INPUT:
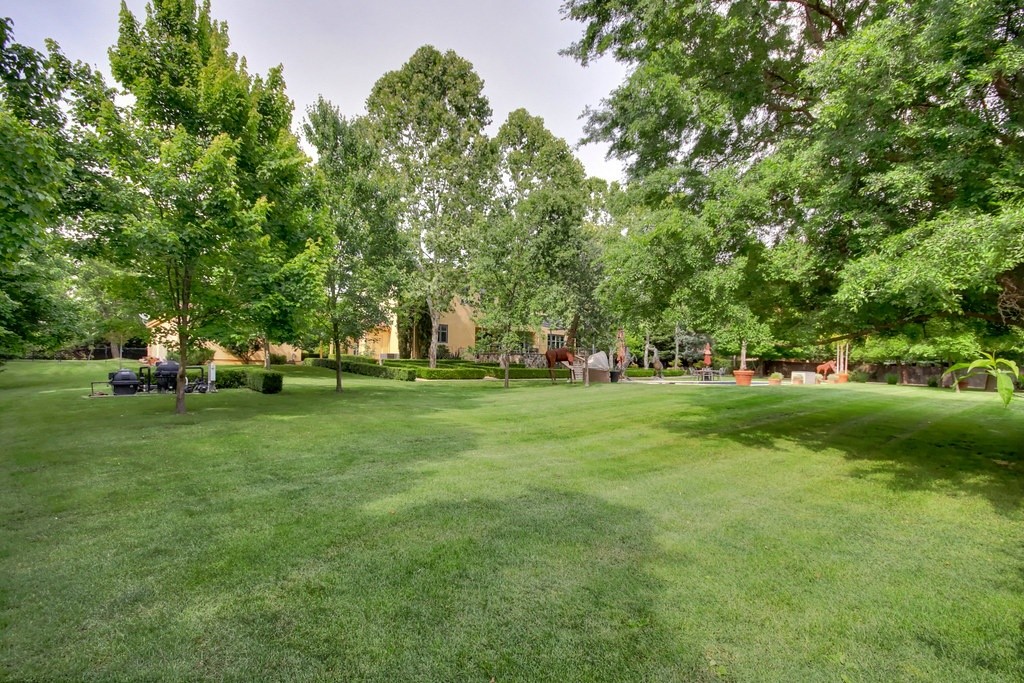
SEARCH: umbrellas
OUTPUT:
[704,343,712,368]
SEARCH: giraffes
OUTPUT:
[546,326,577,382]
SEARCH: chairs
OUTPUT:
[692,367,722,381]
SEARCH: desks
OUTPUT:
[693,370,719,380]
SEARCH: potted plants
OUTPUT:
[793,374,804,385]
[837,369,848,382]
[769,372,784,385]
[815,374,823,384]
[609,364,620,383]
